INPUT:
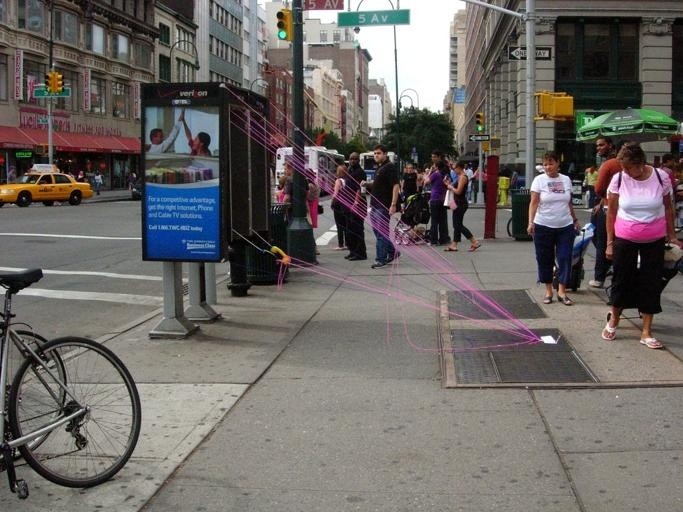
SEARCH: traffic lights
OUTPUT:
[54,72,64,93]
[490,137,501,149]
[45,73,53,94]
[276,8,294,41]
[536,93,552,117]
[475,112,485,133]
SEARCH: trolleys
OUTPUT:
[605,240,682,330]
[553,229,586,294]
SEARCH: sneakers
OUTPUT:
[344,254,366,260]
[333,246,348,249]
[387,250,400,263]
[371,261,384,267]
[589,280,603,287]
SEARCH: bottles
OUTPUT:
[360,183,366,197]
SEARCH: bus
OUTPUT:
[272,145,345,197]
[359,151,398,196]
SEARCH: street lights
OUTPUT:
[249,78,273,98]
[167,40,200,84]
[398,88,420,113]
[353,1,399,123]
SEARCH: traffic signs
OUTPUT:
[467,135,491,142]
[506,44,553,62]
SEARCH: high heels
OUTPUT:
[544,293,553,303]
[557,292,573,305]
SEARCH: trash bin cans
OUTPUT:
[510,189,532,241]
[246,204,289,284]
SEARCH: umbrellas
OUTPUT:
[575,106,682,142]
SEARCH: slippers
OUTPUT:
[467,244,480,251]
[602,311,616,340]
[640,338,663,349]
[444,247,457,251]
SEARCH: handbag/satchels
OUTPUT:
[443,189,454,207]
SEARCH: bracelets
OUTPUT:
[606,241,613,246]
[668,238,678,244]
[573,218,577,223]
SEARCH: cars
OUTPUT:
[130,177,142,201]
[0,163,94,208]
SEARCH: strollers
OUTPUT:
[394,182,434,246]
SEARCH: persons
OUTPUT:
[0,163,139,206]
[527,149,584,307]
[601,141,682,351]
[583,138,683,288]
[179,108,218,158]
[146,108,179,158]
[330,145,485,268]
[500,164,519,189]
[277,160,317,229]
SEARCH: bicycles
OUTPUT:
[1,311,67,464]
[0,268,142,499]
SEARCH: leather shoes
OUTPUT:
[606,287,612,305]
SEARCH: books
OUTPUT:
[146,163,216,185]
